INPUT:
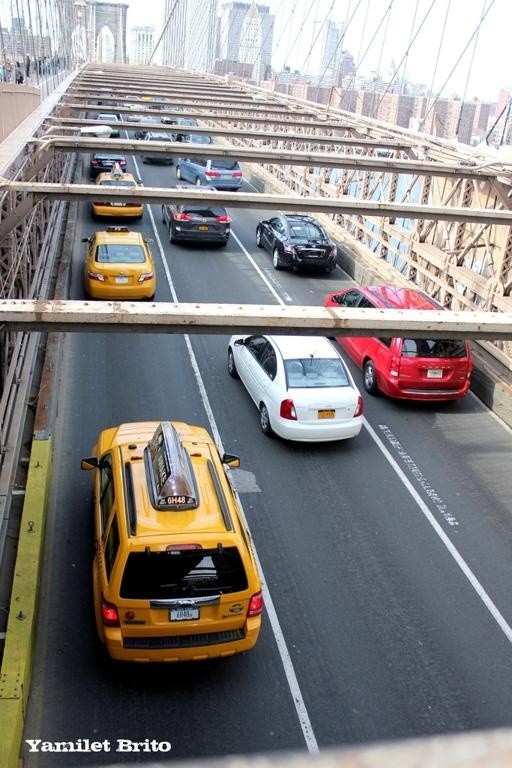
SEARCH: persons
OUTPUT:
[0,52,60,85]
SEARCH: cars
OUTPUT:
[256,215,337,273]
[91,93,243,245]
[81,226,156,301]
[81,421,262,663]
[228,285,474,443]
